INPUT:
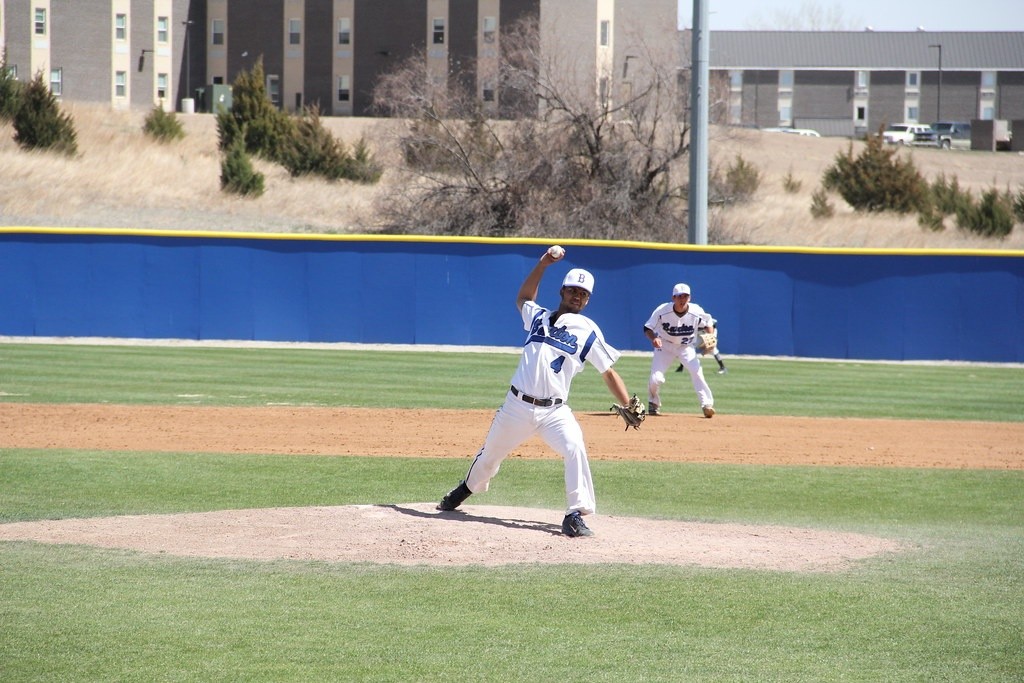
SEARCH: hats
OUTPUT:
[561,269,595,294]
[672,283,690,296]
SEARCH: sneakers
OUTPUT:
[562,511,596,539]
[440,481,471,511]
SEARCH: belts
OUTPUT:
[511,385,563,407]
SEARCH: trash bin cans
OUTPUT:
[205,84,233,114]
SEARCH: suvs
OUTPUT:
[913,121,972,152]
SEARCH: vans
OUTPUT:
[871,122,932,146]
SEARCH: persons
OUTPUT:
[675,317,726,373]
[437,249,645,538]
[644,283,716,418]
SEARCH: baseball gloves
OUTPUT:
[698,333,717,356]
[609,393,646,431]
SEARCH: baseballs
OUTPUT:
[551,245,562,258]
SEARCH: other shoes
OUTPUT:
[702,405,714,418]
[648,401,660,415]
[717,368,727,374]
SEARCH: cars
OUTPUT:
[761,127,820,137]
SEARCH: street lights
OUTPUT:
[182,20,194,98]
[928,43,942,123]
